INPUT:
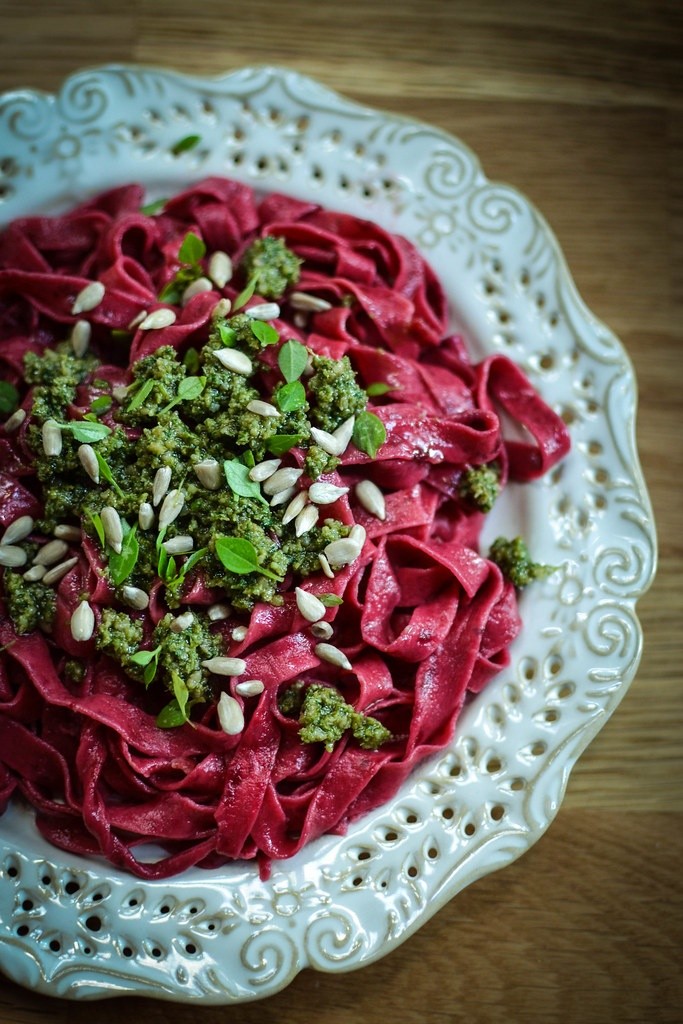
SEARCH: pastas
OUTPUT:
[0,178,572,877]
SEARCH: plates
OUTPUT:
[0,62,658,1000]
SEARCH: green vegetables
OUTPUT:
[54,201,397,728]
